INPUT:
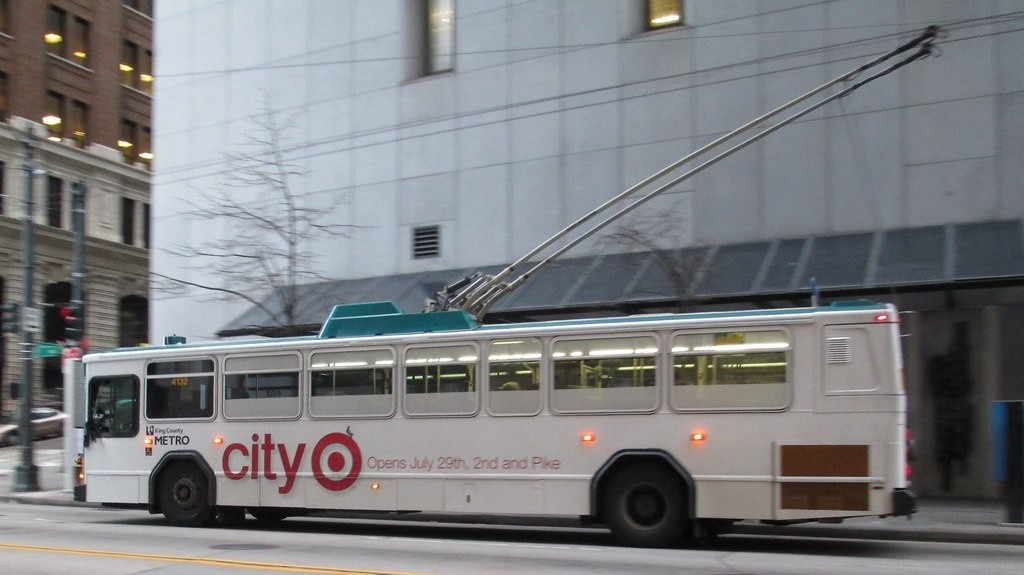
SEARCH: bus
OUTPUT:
[70,24,944,551]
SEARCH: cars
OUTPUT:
[0,407,65,449]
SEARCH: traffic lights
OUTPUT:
[59,304,85,343]
[0,302,18,335]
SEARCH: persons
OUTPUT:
[924,344,974,495]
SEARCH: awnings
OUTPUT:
[213,214,1024,341]
